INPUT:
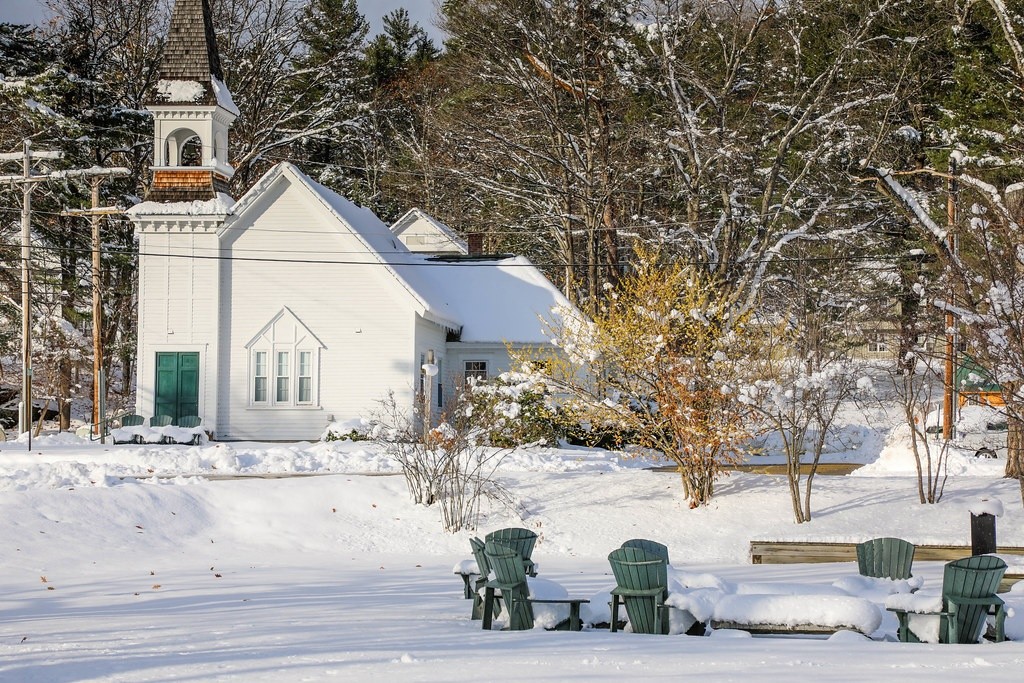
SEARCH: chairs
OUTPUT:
[621,539,669,567]
[483,542,590,631]
[169,416,201,445]
[842,537,921,596]
[610,547,677,635]
[112,416,144,443]
[140,415,172,443]
[888,556,1008,644]
[454,537,501,619]
[484,528,537,577]
